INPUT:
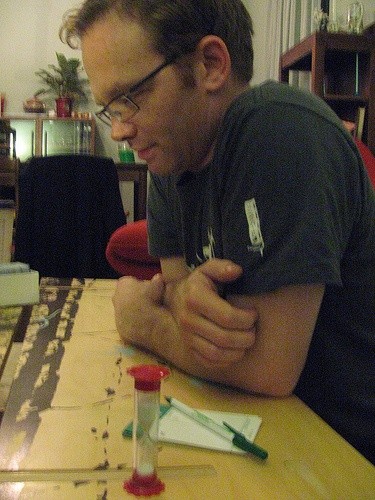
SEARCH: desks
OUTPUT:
[0,277,375,500]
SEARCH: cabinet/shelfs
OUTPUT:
[277,29,368,142]
[0,111,149,263]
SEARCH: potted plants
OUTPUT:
[34,52,89,117]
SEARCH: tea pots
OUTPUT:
[21,96,47,113]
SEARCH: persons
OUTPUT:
[59,0,375,466]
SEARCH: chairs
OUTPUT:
[14,152,126,279]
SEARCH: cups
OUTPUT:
[71,111,89,119]
[118,142,134,164]
[347,1,363,35]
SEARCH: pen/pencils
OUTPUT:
[164,394,269,461]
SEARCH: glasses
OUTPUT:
[94,59,173,127]
